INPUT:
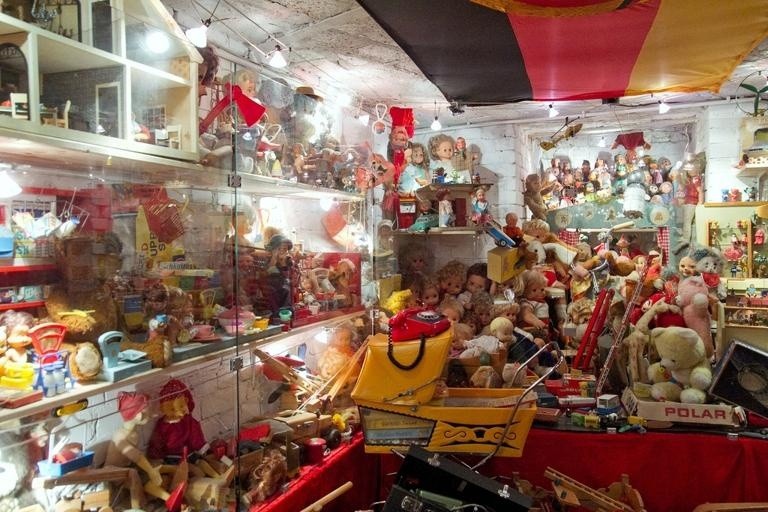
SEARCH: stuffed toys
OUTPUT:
[0,45,767,512]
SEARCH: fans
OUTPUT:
[735,70,768,116]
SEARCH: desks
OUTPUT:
[379,411,768,512]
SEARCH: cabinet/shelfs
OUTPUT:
[704,164,768,365]
[393,182,492,263]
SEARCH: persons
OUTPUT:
[0,45,767,512]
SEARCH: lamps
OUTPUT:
[354,111,371,128]
[656,98,671,115]
[596,136,608,150]
[431,101,442,131]
[184,1,224,49]
[267,43,288,69]
[548,102,560,119]
